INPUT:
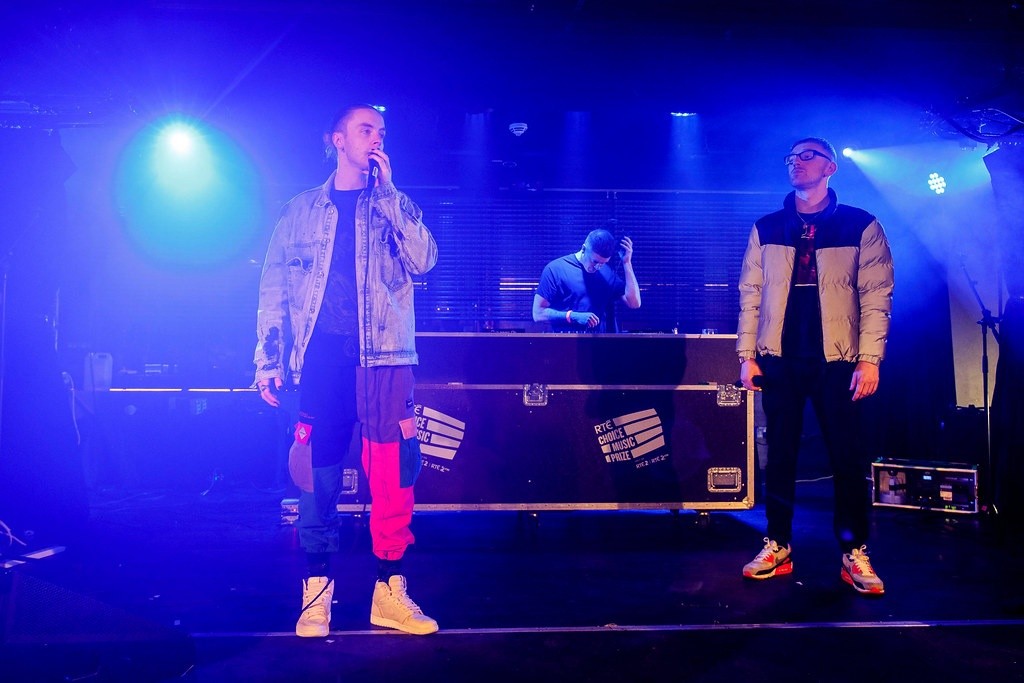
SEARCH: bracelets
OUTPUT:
[566,311,573,323]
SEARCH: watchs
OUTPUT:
[739,356,753,364]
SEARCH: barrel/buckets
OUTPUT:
[84,351,113,391]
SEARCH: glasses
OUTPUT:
[784,150,832,167]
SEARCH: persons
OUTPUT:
[249,104,439,638]
[532,229,641,333]
[735,136,895,594]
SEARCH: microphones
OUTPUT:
[735,376,763,387]
[366,153,380,200]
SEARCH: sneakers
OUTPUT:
[743,537,794,580]
[369,574,439,636]
[840,545,885,595]
[294,575,333,637]
